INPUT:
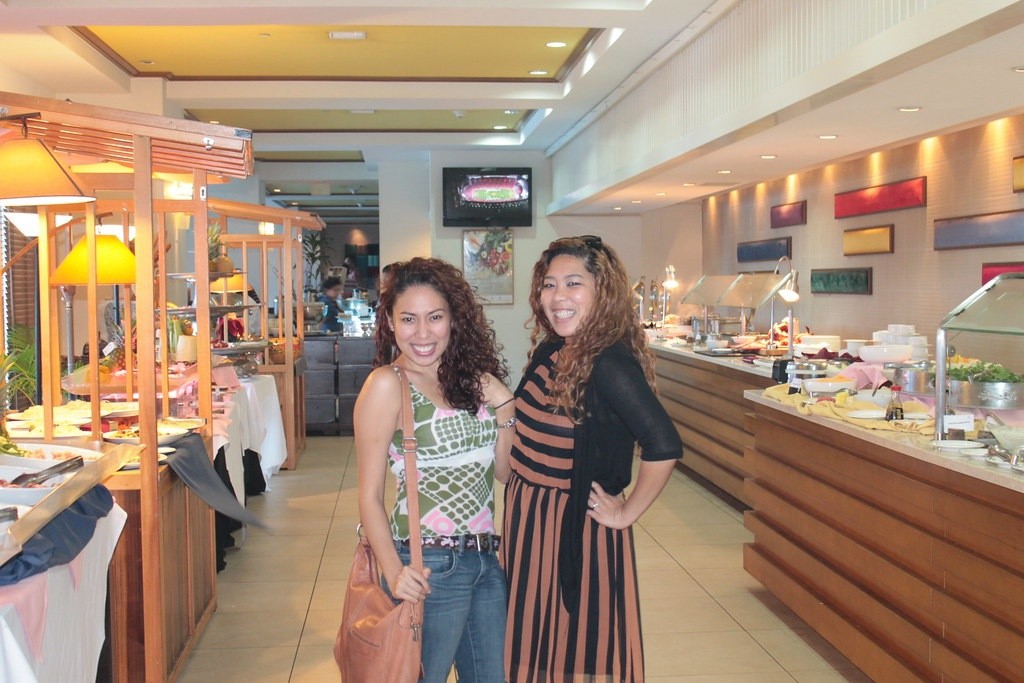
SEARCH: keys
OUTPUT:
[410,621,420,642]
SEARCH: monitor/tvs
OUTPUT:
[442,167,533,227]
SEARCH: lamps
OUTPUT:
[2,210,136,286]
[258,221,275,235]
[209,270,253,294]
[767,255,800,349]
[0,111,97,206]
[662,264,679,332]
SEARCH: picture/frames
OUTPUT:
[462,229,514,305]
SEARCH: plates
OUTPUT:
[157,446,176,453]
[931,439,1024,471]
[802,390,932,420]
[122,453,168,469]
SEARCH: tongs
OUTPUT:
[988,443,1020,464]
[162,417,207,428]
[12,455,85,486]
[0,508,18,524]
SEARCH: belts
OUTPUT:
[400,532,501,552]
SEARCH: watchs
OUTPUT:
[497,417,516,428]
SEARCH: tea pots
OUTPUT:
[355,303,373,317]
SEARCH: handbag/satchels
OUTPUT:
[333,536,425,683]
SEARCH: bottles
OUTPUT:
[885,386,904,420]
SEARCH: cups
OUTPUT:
[269,318,286,337]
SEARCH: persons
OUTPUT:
[352,258,517,683]
[504,235,685,683]
[315,276,345,333]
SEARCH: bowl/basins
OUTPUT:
[641,315,929,392]
[0,400,204,530]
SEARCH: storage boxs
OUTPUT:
[302,336,377,424]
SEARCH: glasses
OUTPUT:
[554,234,605,253]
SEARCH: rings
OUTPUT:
[592,504,600,509]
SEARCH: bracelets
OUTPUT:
[494,398,515,411]
